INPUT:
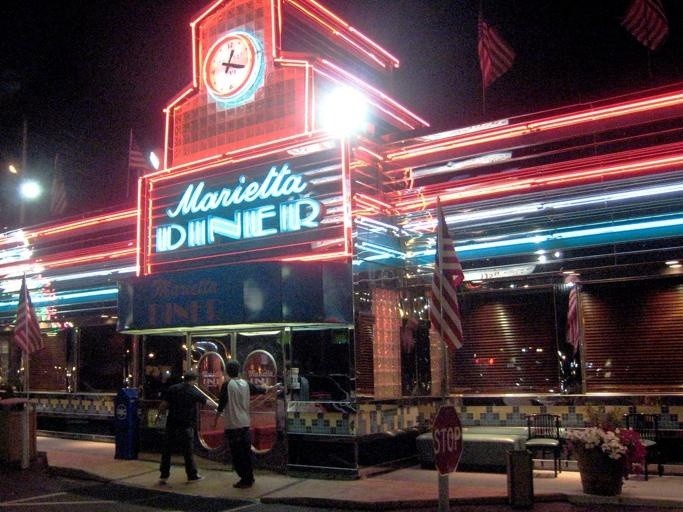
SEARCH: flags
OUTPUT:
[429,203,466,351]
[618,0,670,52]
[476,11,515,89]
[564,282,582,358]
[128,130,153,176]
[12,275,45,357]
[48,159,68,219]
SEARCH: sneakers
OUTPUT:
[232,476,257,489]
[157,472,206,486]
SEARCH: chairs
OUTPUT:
[523,414,563,478]
[623,413,664,480]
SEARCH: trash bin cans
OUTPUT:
[111,387,141,460]
[0,398,38,470]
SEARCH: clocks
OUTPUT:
[200,31,266,108]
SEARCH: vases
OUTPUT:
[575,441,623,497]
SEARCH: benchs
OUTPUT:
[416,434,524,472]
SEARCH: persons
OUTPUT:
[156,370,218,485]
[258,364,285,401]
[279,358,311,402]
[211,360,274,488]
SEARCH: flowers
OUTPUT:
[562,414,648,480]
